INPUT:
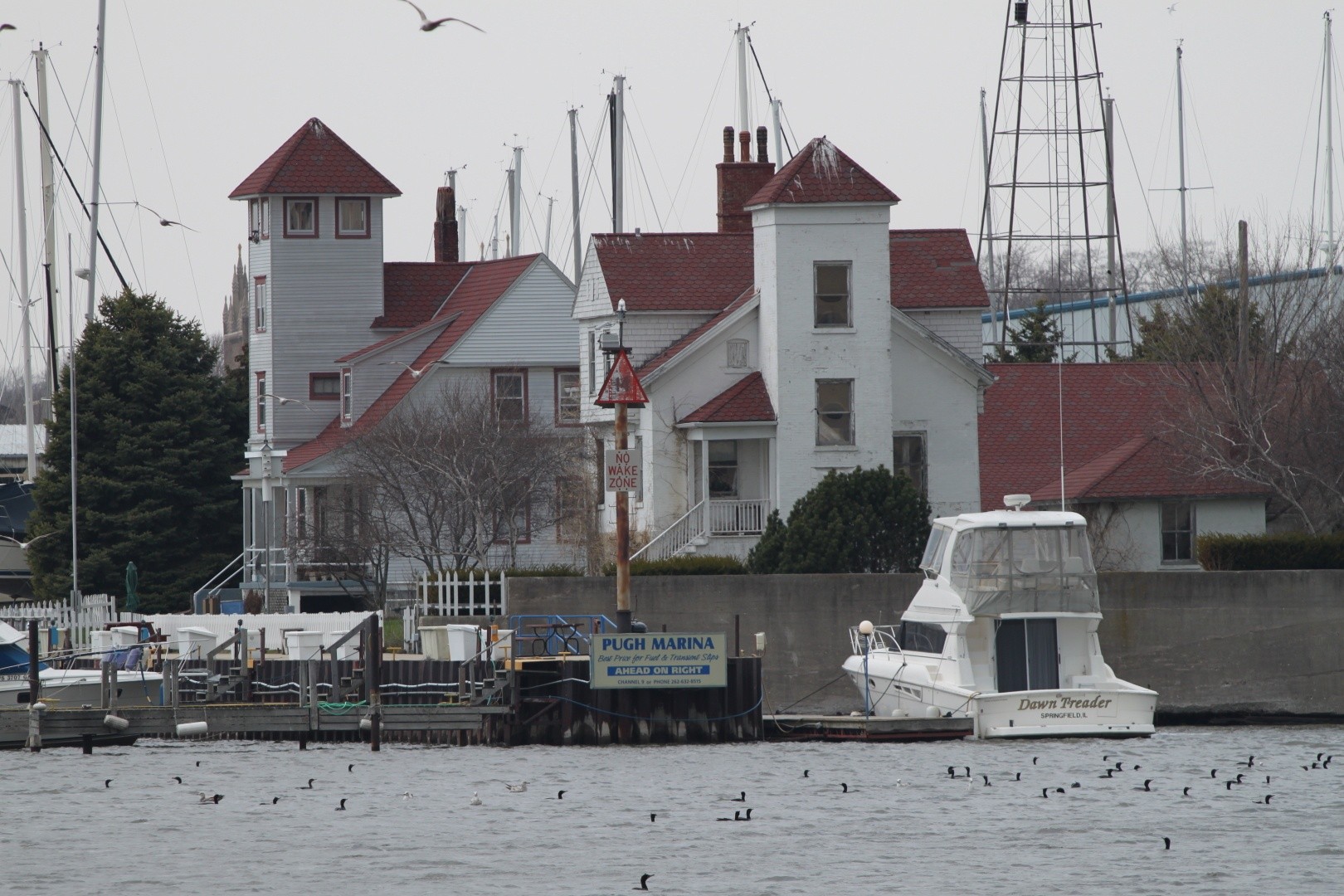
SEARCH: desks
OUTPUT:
[526,624,585,657]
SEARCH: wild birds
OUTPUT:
[1,731,1343,895]
[245,392,311,412]
[401,0,484,34]
[375,360,450,380]
[0,526,66,551]
[137,204,197,233]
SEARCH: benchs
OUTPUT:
[516,637,548,657]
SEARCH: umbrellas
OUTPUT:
[125,562,140,612]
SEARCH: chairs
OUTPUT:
[1058,556,1087,588]
[1013,556,1041,588]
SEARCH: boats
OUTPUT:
[0,617,211,748]
[838,492,1162,736]
